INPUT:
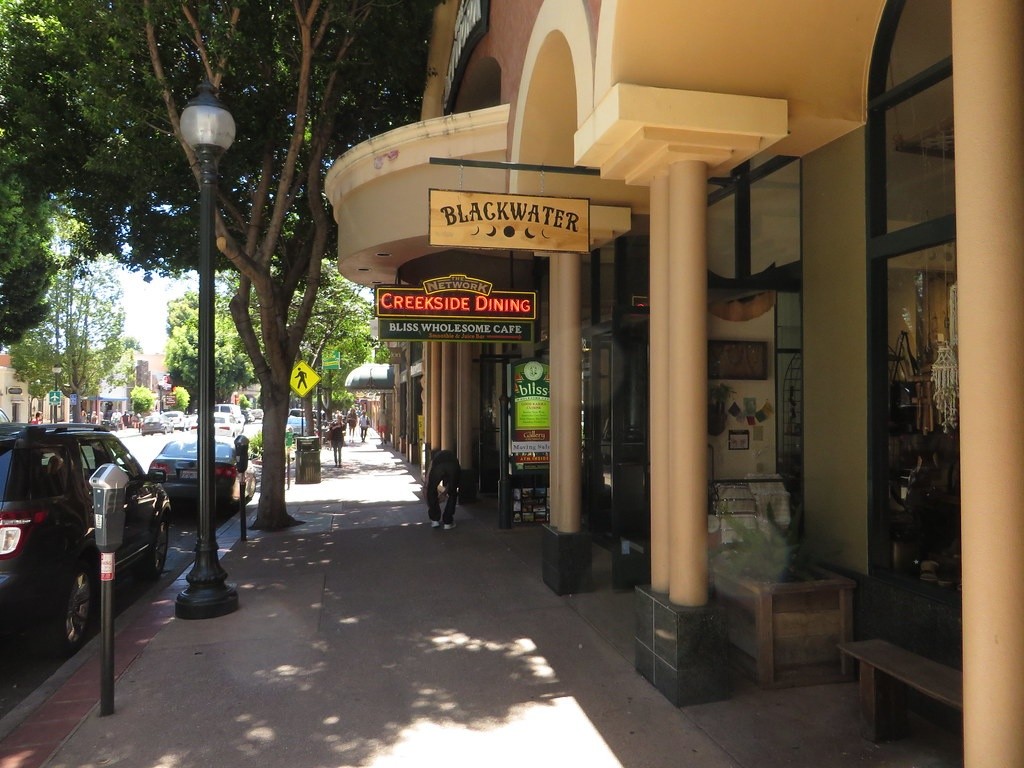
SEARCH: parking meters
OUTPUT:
[286,425,293,490]
[234,435,250,542]
[89,462,131,717]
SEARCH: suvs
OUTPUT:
[0,424,172,653]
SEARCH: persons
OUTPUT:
[82,410,98,424]
[347,408,371,442]
[378,412,388,444]
[100,408,130,430]
[330,415,346,468]
[425,450,461,529]
[32,412,42,424]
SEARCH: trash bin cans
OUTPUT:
[295,437,321,484]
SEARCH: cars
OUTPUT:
[215,403,264,437]
[142,411,198,436]
[287,409,317,439]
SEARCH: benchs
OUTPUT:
[834,639,963,743]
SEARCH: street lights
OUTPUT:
[158,378,165,414]
[52,364,63,423]
[174,79,240,619]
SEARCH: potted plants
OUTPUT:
[709,383,737,416]
[708,495,857,691]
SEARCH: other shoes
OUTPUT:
[444,523,456,530]
[431,521,440,527]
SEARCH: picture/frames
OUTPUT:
[707,339,768,380]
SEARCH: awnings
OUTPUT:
[345,367,395,390]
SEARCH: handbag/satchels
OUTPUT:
[366,417,370,425]
[329,427,333,440]
